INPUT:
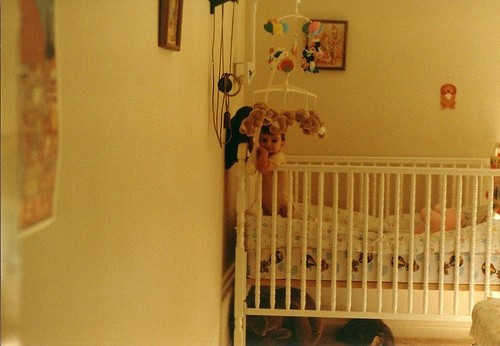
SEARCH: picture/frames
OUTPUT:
[159,0,182,51]
[308,19,348,70]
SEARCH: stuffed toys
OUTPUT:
[248,287,323,345]
[239,103,326,139]
[419,205,464,234]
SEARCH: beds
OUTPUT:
[232,143,500,346]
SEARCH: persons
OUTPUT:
[259,126,294,217]
